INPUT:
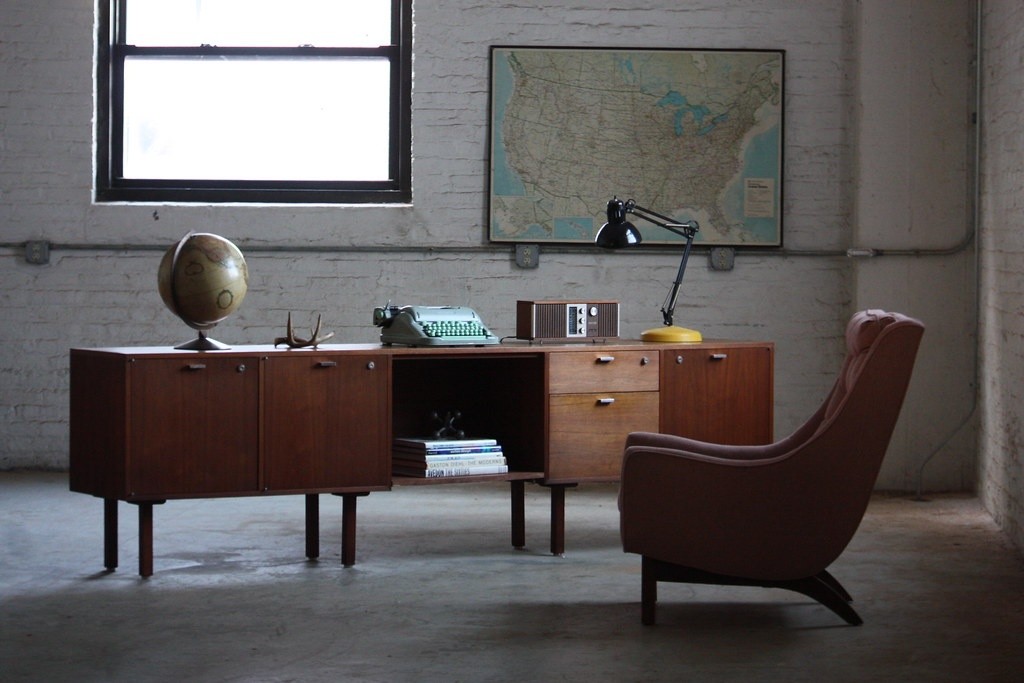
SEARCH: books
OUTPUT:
[392,438,508,478]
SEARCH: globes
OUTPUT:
[156,228,249,351]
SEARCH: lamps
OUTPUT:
[596,196,702,342]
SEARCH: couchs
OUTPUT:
[617,308,926,627]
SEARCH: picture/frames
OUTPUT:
[488,45,785,247]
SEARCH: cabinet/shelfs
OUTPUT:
[69,346,259,501]
[545,339,660,482]
[660,339,775,446]
[261,345,391,494]
